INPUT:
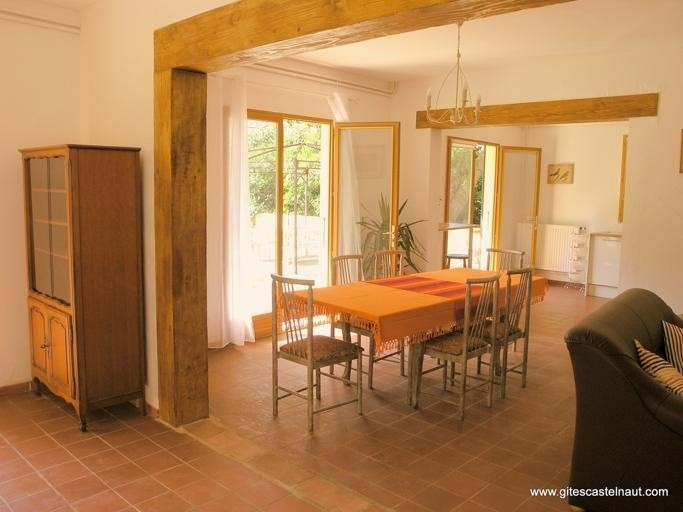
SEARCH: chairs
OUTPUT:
[405,273,503,421]
[486,246,522,272]
[565,287,682,510]
[268,272,366,431]
[374,251,406,280]
[331,254,406,388]
[449,267,532,399]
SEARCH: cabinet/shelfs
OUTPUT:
[17,144,148,432]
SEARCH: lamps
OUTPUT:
[423,13,485,130]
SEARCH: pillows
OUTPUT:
[657,318,683,372]
[631,333,682,396]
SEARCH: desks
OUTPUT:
[443,254,468,266]
[277,265,548,416]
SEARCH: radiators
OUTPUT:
[517,223,581,271]
